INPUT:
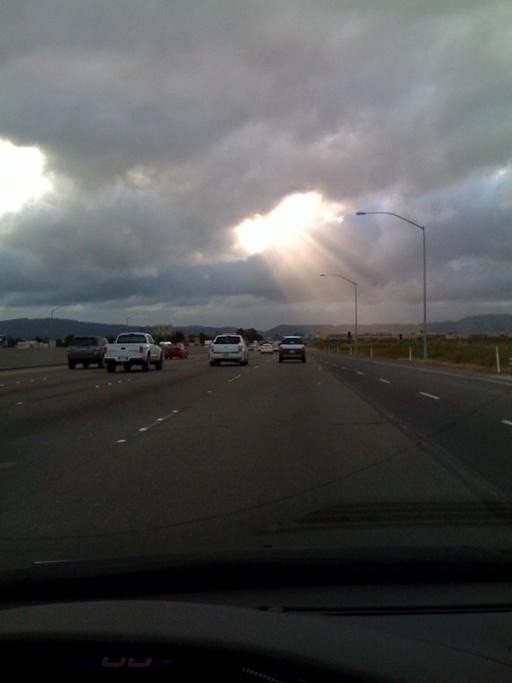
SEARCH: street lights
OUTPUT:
[49,302,78,338]
[318,272,359,342]
[354,210,429,361]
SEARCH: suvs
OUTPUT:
[279,335,307,363]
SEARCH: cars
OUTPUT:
[162,341,190,359]
[158,340,172,347]
[248,337,282,354]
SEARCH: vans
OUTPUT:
[66,334,111,370]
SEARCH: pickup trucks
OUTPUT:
[103,331,163,372]
[207,333,250,366]
